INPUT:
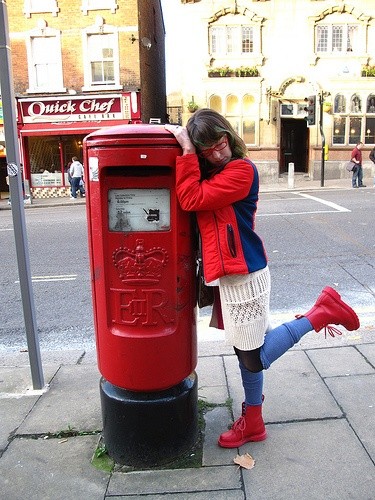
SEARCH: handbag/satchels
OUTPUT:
[346,162,355,172]
[197,261,215,308]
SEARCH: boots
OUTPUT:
[295,286,361,335]
[217,394,267,448]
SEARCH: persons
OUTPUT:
[164,108,360,448]
[369,147,375,163]
[350,142,366,188]
[67,156,86,200]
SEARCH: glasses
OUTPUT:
[198,136,228,158]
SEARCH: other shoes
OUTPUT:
[81,192,86,197]
[352,184,358,188]
[358,184,366,187]
[70,194,78,200]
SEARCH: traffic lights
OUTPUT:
[303,95,317,126]
